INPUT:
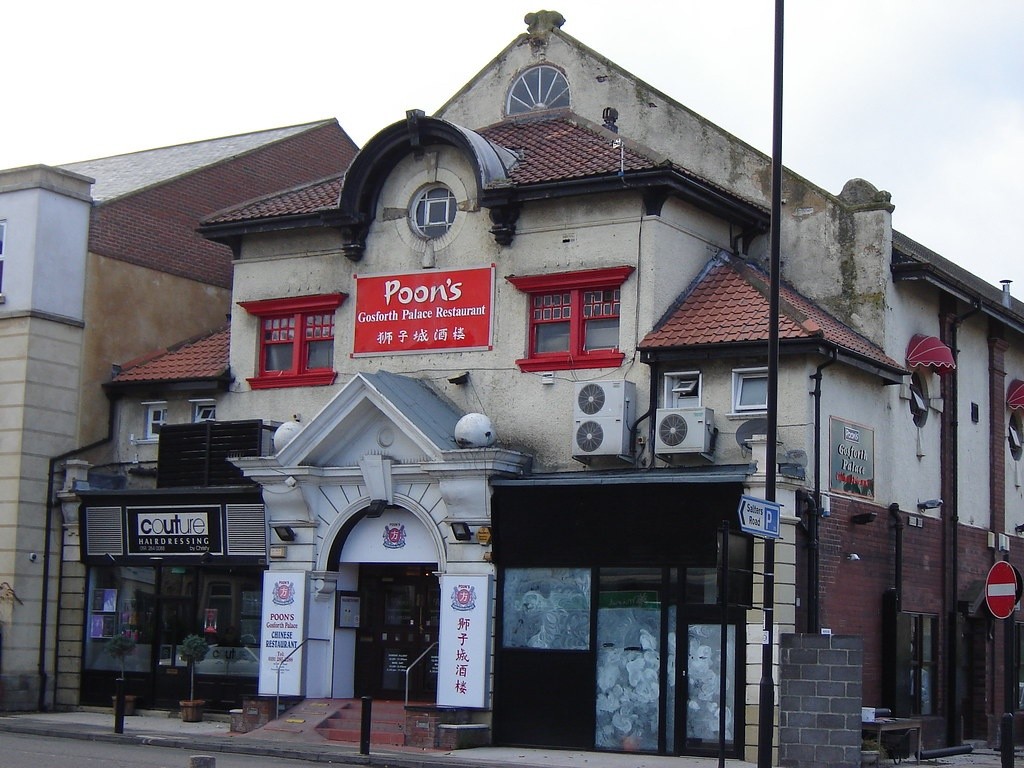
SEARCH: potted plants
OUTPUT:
[178,633,210,722]
[861,740,888,768]
[106,634,137,716]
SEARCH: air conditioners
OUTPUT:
[655,406,715,454]
[571,379,636,458]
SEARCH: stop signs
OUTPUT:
[984,561,1017,619]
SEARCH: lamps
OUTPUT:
[366,499,388,518]
[849,512,878,526]
[272,525,296,541]
[450,521,474,541]
[448,371,470,384]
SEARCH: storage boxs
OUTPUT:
[862,707,876,722]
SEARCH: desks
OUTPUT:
[861,718,923,768]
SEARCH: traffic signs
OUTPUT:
[737,495,781,539]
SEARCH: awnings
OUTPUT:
[909,331,957,376]
[1009,376,1024,409]
